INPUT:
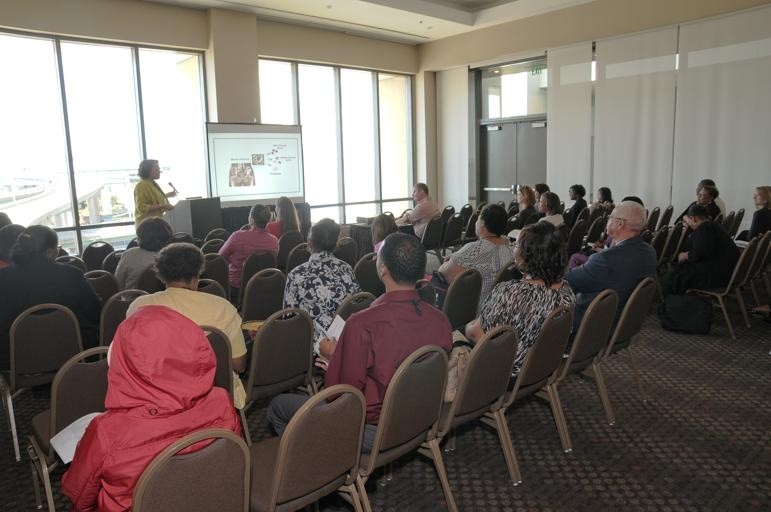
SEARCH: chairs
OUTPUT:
[498,200,505,211]
[198,278,225,301]
[442,268,483,333]
[651,225,669,264]
[567,220,587,255]
[507,206,519,220]
[239,249,278,309]
[53,254,87,274]
[591,207,601,220]
[100,249,126,275]
[99,289,148,359]
[1,303,86,462]
[585,217,607,247]
[729,208,745,239]
[279,230,304,271]
[491,260,523,290]
[352,344,458,512]
[684,236,759,339]
[754,239,771,299]
[646,207,661,232]
[243,267,285,321]
[251,383,364,512]
[333,237,358,268]
[640,229,654,243]
[81,240,114,270]
[312,292,376,396]
[84,269,121,305]
[132,427,250,512]
[735,230,769,330]
[537,289,613,451]
[655,222,684,304]
[478,201,488,215]
[463,210,481,247]
[137,264,167,295]
[413,278,437,309]
[238,308,313,446]
[562,208,576,228]
[723,210,735,235]
[441,212,464,261]
[168,231,194,245]
[508,199,521,212]
[197,325,234,409]
[506,215,521,237]
[200,239,225,254]
[460,204,473,231]
[26,345,109,512]
[204,228,230,244]
[595,278,657,424]
[441,205,455,231]
[432,325,519,487]
[672,226,692,264]
[575,207,591,225]
[354,251,379,295]
[658,205,675,231]
[284,241,310,279]
[127,237,142,249]
[487,305,570,453]
[421,216,443,265]
[199,252,232,303]
[561,201,565,213]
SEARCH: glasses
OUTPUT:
[513,242,520,249]
[53,246,62,251]
[608,215,624,222]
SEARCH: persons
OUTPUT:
[0,212,26,268]
[661,204,740,292]
[266,198,301,238]
[674,187,720,225]
[51,306,241,511]
[398,182,443,240]
[568,196,644,269]
[696,180,726,223]
[436,202,518,318]
[1,225,105,371]
[563,201,656,350]
[123,242,247,412]
[371,212,398,250]
[508,190,563,241]
[736,187,771,241]
[133,159,177,233]
[466,221,575,391]
[114,217,176,300]
[590,187,613,220]
[509,187,537,227]
[217,205,280,304]
[565,185,588,224]
[533,185,551,208]
[282,217,363,365]
[268,233,453,493]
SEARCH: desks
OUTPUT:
[357,217,418,250]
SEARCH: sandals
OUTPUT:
[746,308,771,322]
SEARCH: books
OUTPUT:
[51,410,112,464]
[315,313,345,369]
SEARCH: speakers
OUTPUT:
[169,182,179,193]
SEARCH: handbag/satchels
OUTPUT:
[660,294,714,333]
[444,329,474,402]
